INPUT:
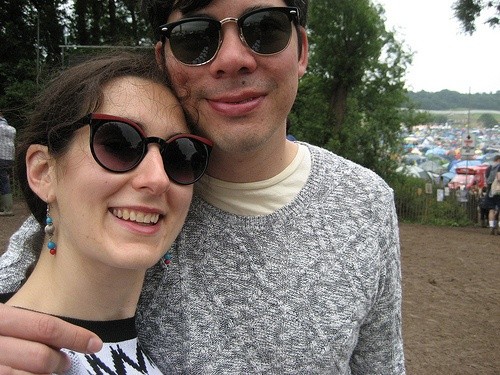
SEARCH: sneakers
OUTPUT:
[490,225,494,235]
[495,227,500,234]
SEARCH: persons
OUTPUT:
[0,0,407,375]
[1,51,213,375]
[466,155,500,236]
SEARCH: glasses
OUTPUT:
[48,111,215,187]
[156,6,301,68]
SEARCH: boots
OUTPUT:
[0,194,14,215]
